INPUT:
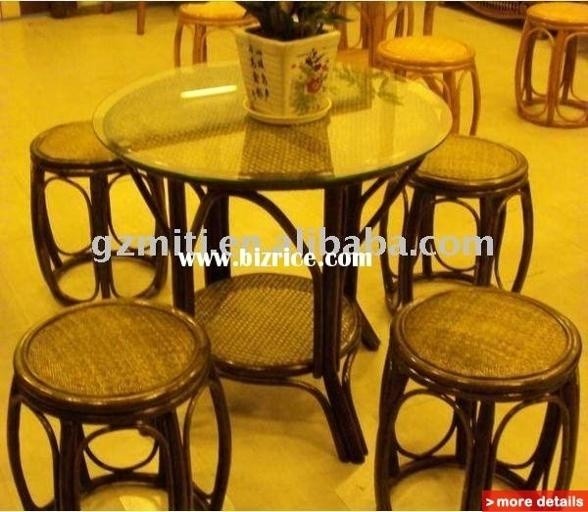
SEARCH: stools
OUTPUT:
[6,1,588,511]
